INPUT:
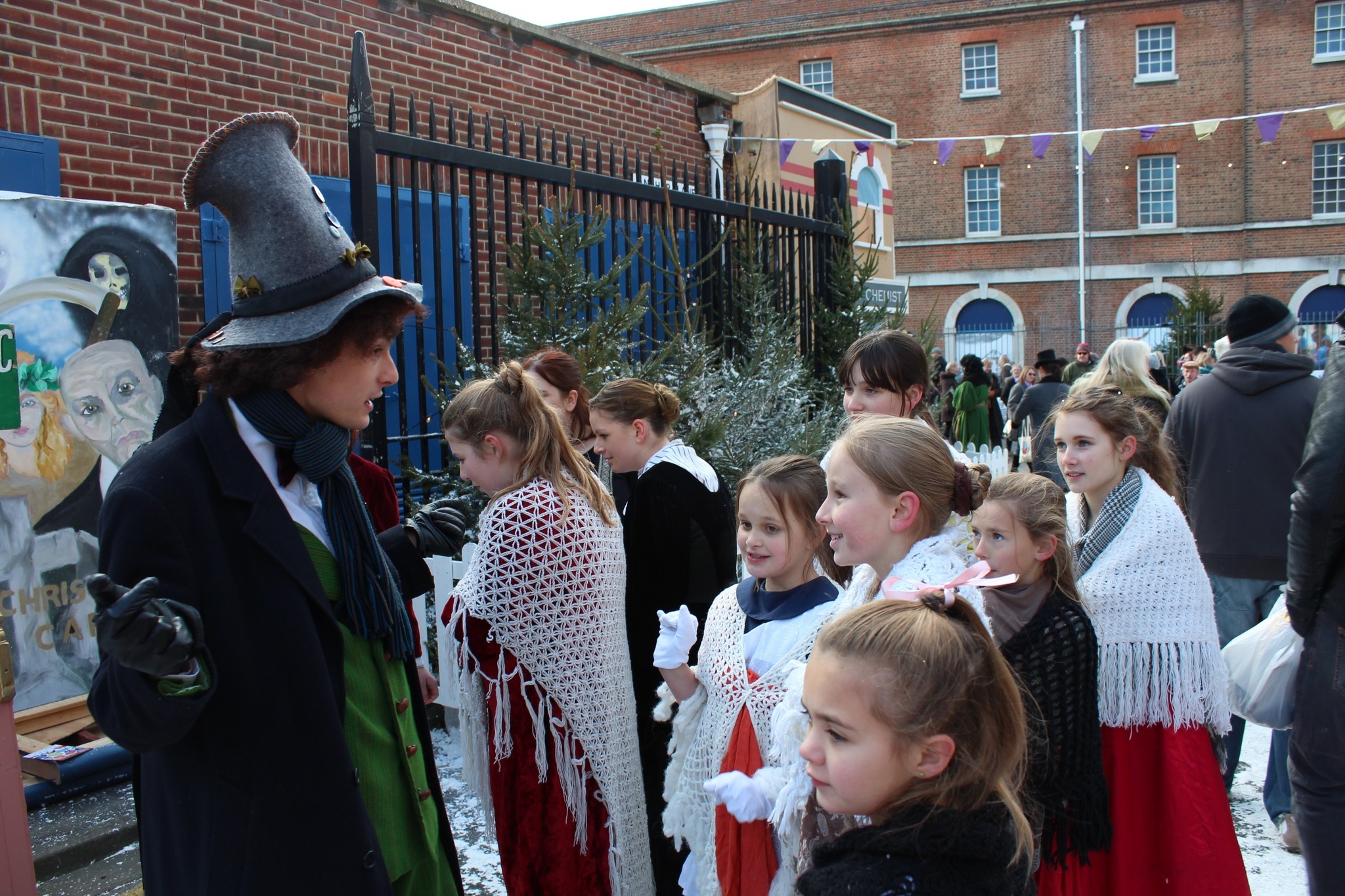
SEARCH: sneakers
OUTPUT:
[1280,815,1301,852]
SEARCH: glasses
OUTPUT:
[1027,373,1036,376]
[1077,352,1088,355]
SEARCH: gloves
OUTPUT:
[406,494,470,559]
[652,605,698,669]
[701,767,785,824]
[86,571,205,676]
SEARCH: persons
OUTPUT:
[839,329,971,464]
[652,455,847,896]
[1069,339,1170,425]
[524,349,639,519]
[928,348,1071,493]
[795,589,1033,896]
[972,473,1112,869]
[440,360,659,896]
[84,298,467,895]
[1162,295,1322,851]
[1036,385,1253,896]
[1283,311,1344,896]
[586,379,737,896]
[815,415,990,638]
[1062,343,1096,386]
[1150,351,1169,392]
[1175,343,1218,390]
[1297,324,1332,370]
[347,452,439,705]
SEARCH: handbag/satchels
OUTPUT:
[996,396,1008,425]
[1002,419,1019,440]
[1019,415,1032,464]
[1217,592,1304,730]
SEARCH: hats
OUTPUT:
[1148,354,1160,370]
[931,348,942,353]
[1183,345,1196,353]
[1226,295,1299,348]
[1193,347,1207,355]
[1034,349,1060,369]
[182,112,423,351]
[1077,344,1091,352]
[1182,361,1200,369]
